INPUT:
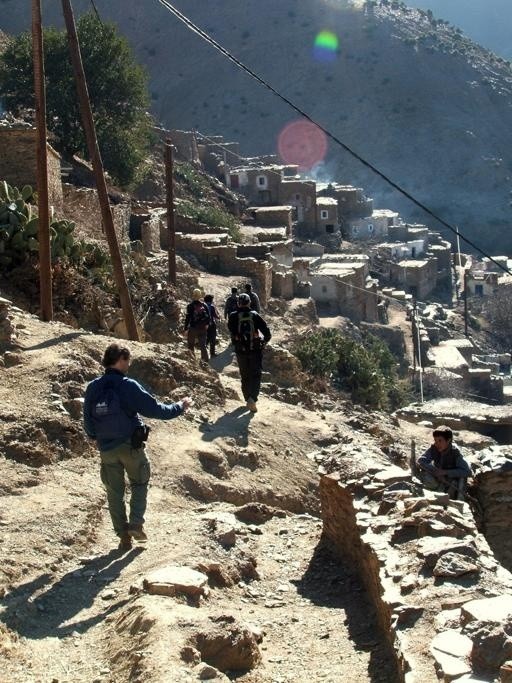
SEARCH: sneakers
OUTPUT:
[120,537,133,550]
[246,396,258,412]
[127,523,147,540]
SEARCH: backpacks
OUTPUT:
[89,377,136,441]
[231,311,265,352]
[192,302,211,325]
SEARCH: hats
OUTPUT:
[238,292,251,305]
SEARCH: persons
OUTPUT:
[417,424,472,496]
[80,341,197,547]
[204,294,220,355]
[244,282,262,314]
[227,293,272,414]
[184,289,209,361]
[224,286,240,318]
